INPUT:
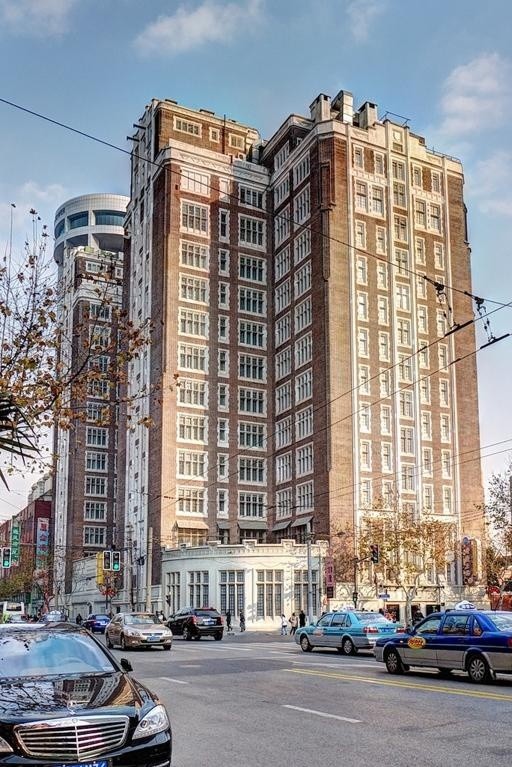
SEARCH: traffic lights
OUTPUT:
[353,591,358,602]
[103,550,112,570]
[113,550,121,571]
[369,543,378,563]
[3,546,12,568]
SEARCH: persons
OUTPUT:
[380,607,425,627]
[280,613,288,635]
[299,609,306,627]
[76,612,83,623]
[226,611,233,631]
[239,611,245,631]
[289,612,298,635]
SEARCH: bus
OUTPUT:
[1,601,26,623]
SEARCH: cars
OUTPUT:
[294,609,409,655]
[105,611,174,649]
[7,613,30,622]
[35,609,69,625]
[81,613,112,632]
[1,620,172,765]
[373,599,512,684]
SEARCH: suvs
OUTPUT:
[168,606,225,640]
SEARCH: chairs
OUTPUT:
[0,637,86,674]
